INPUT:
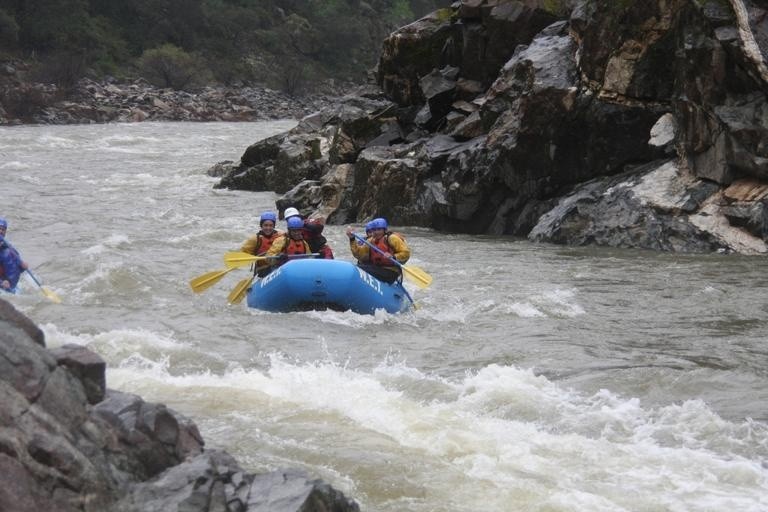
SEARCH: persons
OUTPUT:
[346,218,410,285]
[284,208,304,223]
[356,221,373,246]
[240,212,286,275]
[305,219,334,259]
[0,218,28,293]
[264,217,315,265]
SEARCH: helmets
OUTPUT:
[284,206,300,219]
[260,211,276,224]
[366,218,388,233]
[286,216,304,231]
[304,218,324,236]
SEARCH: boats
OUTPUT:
[246,259,404,314]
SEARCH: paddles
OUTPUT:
[224,251,325,268]
[189,266,237,293]
[349,229,433,289]
[396,276,423,314]
[228,271,258,305]
